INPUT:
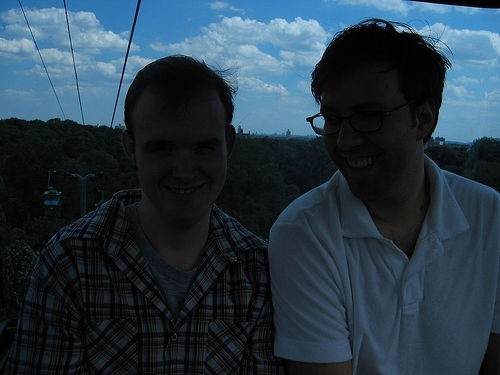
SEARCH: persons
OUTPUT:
[268,20,500,375]
[3,58,284,375]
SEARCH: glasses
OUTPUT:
[307,102,411,136]
